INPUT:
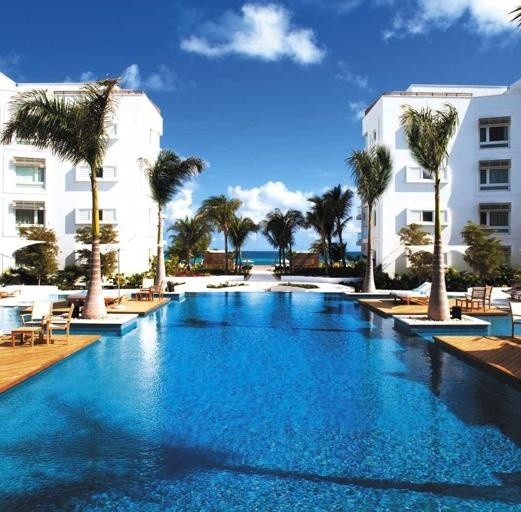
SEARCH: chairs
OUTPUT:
[149,280,165,301]
[508,301,521,338]
[389,282,432,305]
[464,284,493,312]
[18,298,76,348]
